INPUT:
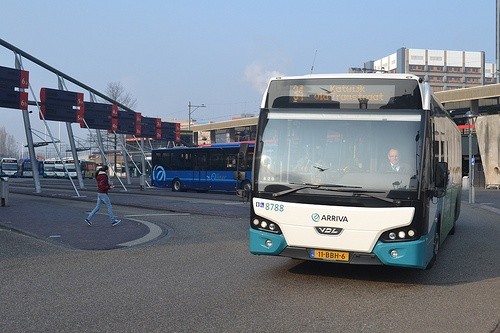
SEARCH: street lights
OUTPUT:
[465,111,476,205]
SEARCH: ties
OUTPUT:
[393,166,397,174]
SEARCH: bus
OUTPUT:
[22,158,45,177]
[0,157,19,177]
[150,141,255,192]
[249,73,463,268]
[43,158,127,179]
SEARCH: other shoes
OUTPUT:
[113,220,121,227]
[85,219,92,227]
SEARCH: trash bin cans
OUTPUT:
[461,176,469,190]
[0,177,8,207]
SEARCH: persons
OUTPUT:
[85,165,121,226]
[380,148,403,174]
